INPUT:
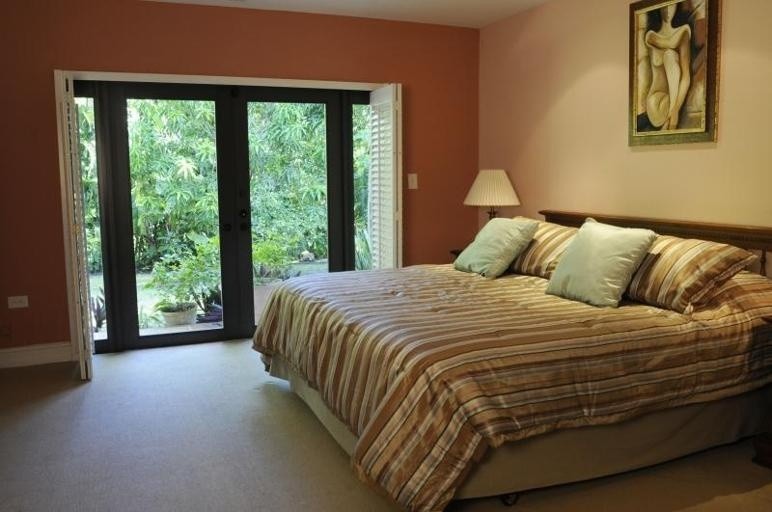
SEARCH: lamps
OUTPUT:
[462,169,521,219]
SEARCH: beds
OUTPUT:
[249,208,772,512]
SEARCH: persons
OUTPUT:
[644,3,691,131]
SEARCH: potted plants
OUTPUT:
[138,261,211,327]
[253,229,302,325]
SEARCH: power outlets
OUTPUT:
[7,295,30,309]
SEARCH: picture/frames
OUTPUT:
[627,0,718,148]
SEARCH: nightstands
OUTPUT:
[761,314,772,322]
[449,248,461,257]
